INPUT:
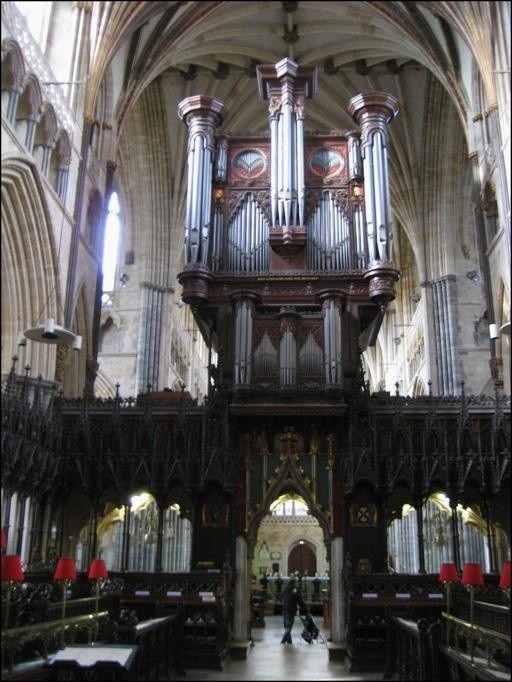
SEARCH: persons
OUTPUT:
[281,579,303,644]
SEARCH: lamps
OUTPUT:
[49,557,78,623]
[18,79,84,353]
[86,558,109,615]
[438,562,458,617]
[488,322,512,339]
[1,553,24,631]
[461,562,488,629]
[498,561,512,597]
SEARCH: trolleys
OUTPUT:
[294,606,331,645]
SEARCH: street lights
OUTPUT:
[299,538,305,572]
